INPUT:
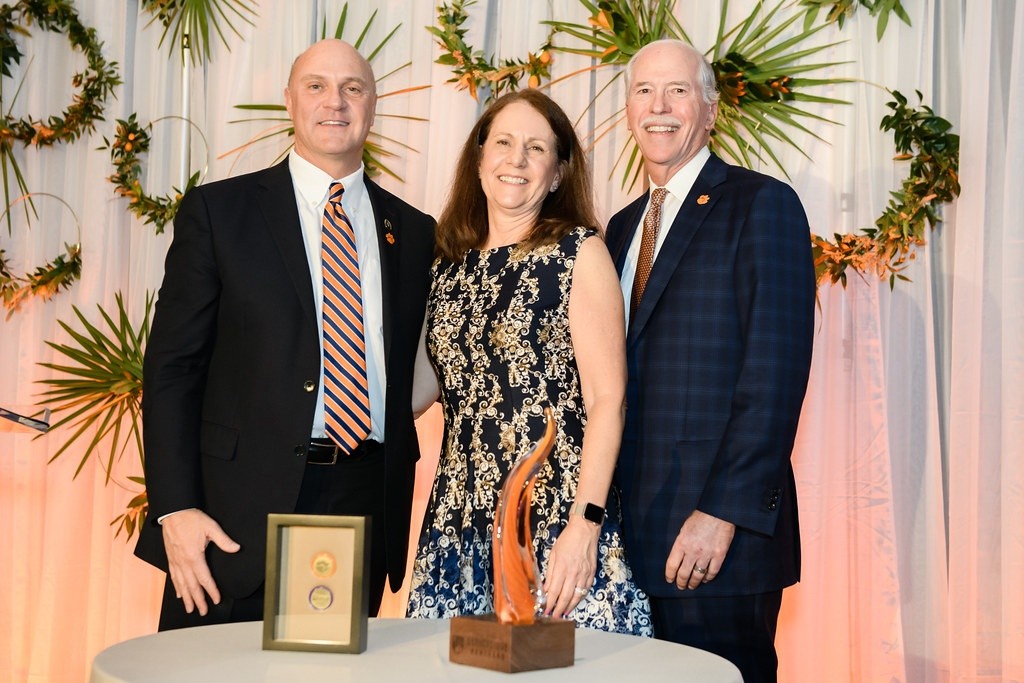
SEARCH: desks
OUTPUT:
[89,618,745,682]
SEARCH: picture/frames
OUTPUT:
[261,513,372,656]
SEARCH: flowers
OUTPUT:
[742,80,962,295]
[0,0,123,240]
[424,0,563,106]
[794,0,912,44]
[1,193,82,323]
[138,0,259,67]
[95,113,209,235]
[536,0,887,197]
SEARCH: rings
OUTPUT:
[575,588,588,595]
[693,565,708,574]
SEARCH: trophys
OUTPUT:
[448,406,576,673]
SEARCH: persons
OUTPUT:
[132,39,441,634]
[601,38,818,683]
[410,89,654,641]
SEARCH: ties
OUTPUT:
[322,182,372,455]
[628,187,670,324]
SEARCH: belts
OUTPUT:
[309,440,371,466]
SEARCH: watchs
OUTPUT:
[568,502,605,527]
